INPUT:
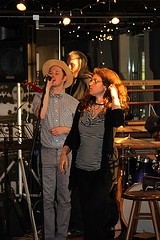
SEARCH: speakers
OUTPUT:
[0,12,31,84]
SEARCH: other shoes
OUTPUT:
[67,229,84,236]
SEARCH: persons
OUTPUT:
[65,51,93,102]
[33,59,81,240]
[59,68,130,240]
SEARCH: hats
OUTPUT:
[42,59,73,88]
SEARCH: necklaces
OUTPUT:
[94,101,104,105]
[94,104,102,112]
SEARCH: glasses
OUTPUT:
[89,78,104,84]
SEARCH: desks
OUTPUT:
[113,120,160,233]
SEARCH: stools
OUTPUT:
[121,190,160,240]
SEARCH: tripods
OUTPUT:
[0,83,45,240]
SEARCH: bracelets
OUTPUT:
[60,153,67,156]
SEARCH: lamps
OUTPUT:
[16,0,26,11]
